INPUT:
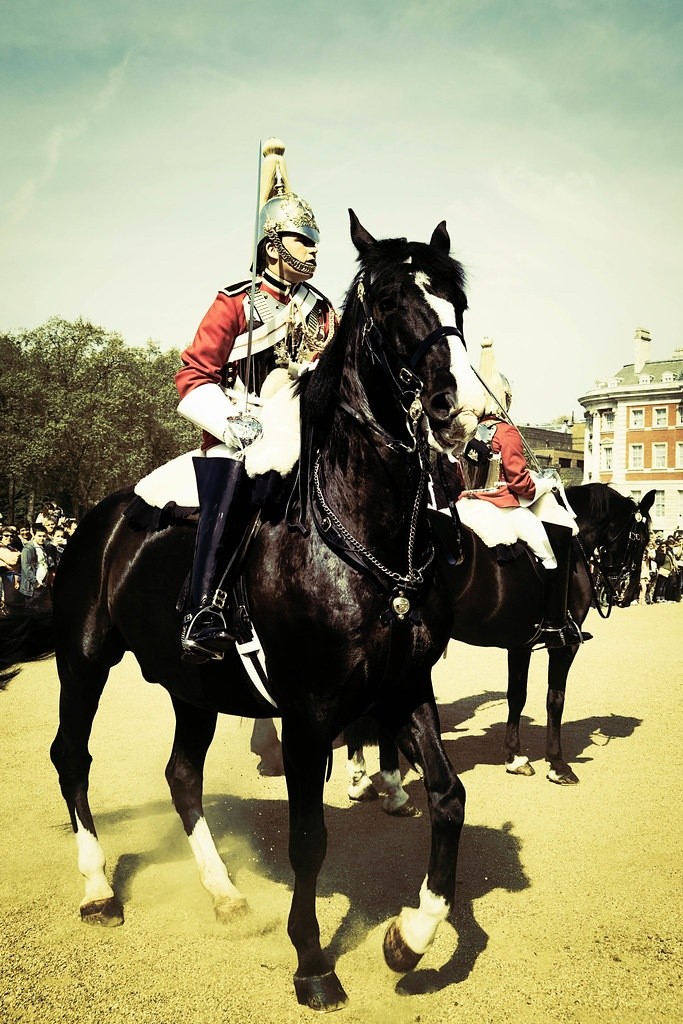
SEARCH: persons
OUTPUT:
[464,350,594,648]
[0,501,78,615]
[177,191,336,649]
[632,529,683,605]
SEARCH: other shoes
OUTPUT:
[630,596,667,606]
[544,620,593,649]
[180,616,236,665]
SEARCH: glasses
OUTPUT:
[661,546,666,548]
[3,534,13,538]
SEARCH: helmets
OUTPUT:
[249,136,321,275]
[474,336,512,416]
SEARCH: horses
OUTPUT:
[0,200,474,1015]
[324,480,660,823]
